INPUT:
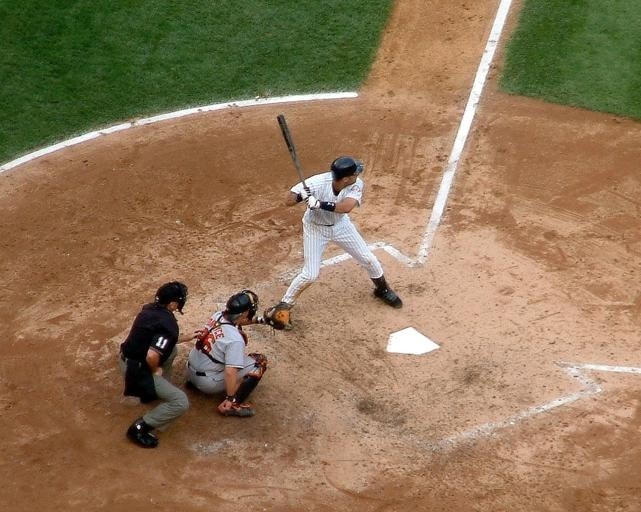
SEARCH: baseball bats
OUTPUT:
[277,115,315,210]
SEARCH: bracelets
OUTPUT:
[256,316,264,324]
[320,201,335,211]
[296,193,303,202]
[224,395,238,402]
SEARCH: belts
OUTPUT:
[324,224,334,226]
[188,362,206,377]
[121,354,141,367]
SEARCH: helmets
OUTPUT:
[154,281,188,309]
[330,156,364,174]
[225,290,260,312]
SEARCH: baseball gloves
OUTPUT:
[264,303,294,330]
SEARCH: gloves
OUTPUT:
[295,187,312,203]
[305,196,320,211]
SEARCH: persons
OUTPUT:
[186,289,275,418]
[119,280,201,448]
[264,156,403,329]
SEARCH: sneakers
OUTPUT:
[126,426,159,448]
[276,305,293,330]
[225,404,256,418]
[373,284,402,309]
